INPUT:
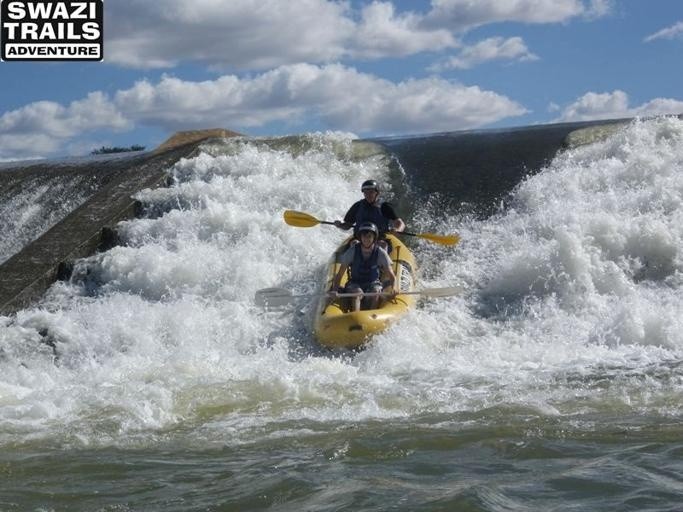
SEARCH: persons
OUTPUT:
[327,222,400,311]
[335,179,406,255]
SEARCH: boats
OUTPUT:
[312,234,418,352]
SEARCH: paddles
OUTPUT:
[255,286,463,306]
[284,210,462,245]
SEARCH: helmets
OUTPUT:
[356,222,379,242]
[362,181,380,193]
